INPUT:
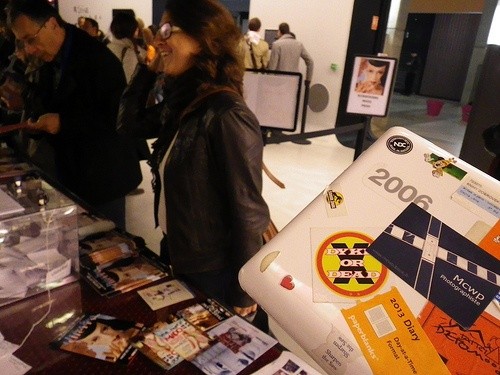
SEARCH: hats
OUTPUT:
[110,9,138,37]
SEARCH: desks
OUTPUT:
[0,135,281,375]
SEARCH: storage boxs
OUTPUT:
[0,178,81,307]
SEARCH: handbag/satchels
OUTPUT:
[262,219,279,245]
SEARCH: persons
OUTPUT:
[266,23,313,143]
[0,0,128,235]
[356,60,386,95]
[75,6,157,196]
[74,319,129,357]
[109,258,151,286]
[239,18,270,70]
[151,0,280,335]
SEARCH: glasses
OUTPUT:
[17,17,48,48]
[156,22,184,40]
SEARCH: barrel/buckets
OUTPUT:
[427,99,444,116]
